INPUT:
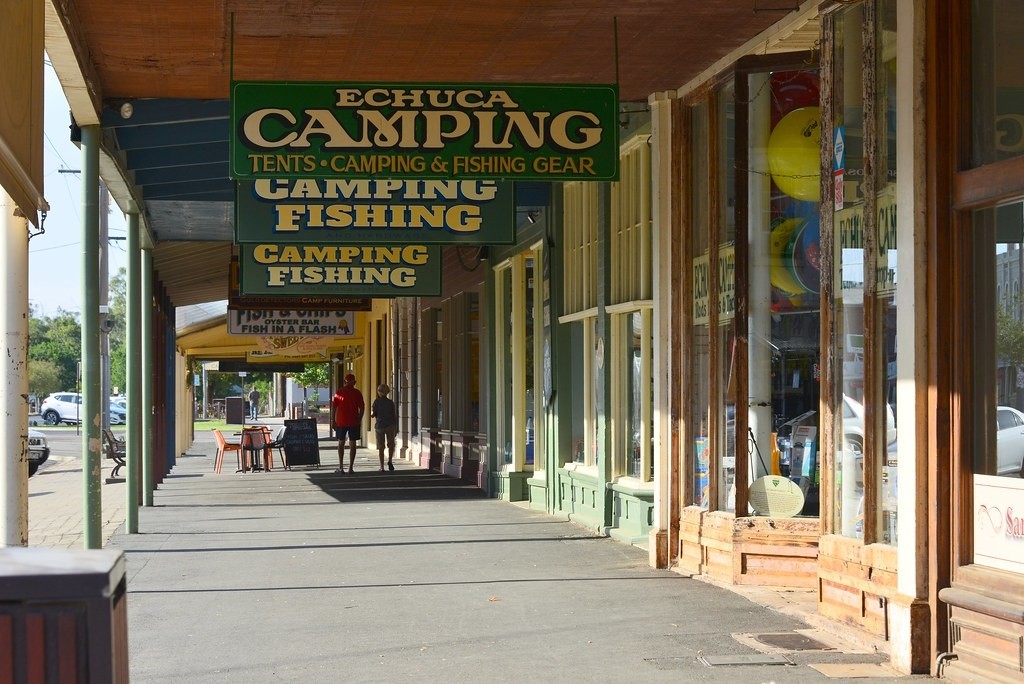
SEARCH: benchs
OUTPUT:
[102,428,126,478]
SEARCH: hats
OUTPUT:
[345,374,356,383]
[378,384,389,394]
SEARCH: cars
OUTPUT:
[28,429,50,479]
[109,396,126,408]
[996,405,1024,477]
[39,392,126,426]
[842,394,896,456]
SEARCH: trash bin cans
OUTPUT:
[1,547,129,684]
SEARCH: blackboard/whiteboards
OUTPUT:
[284,417,320,466]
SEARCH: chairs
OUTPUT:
[211,426,292,474]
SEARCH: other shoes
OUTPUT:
[379,468,385,472]
[388,463,394,470]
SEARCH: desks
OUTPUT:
[232,432,272,473]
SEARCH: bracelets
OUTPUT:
[330,420,334,421]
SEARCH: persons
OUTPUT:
[248,387,260,420]
[330,374,365,474]
[370,384,398,472]
